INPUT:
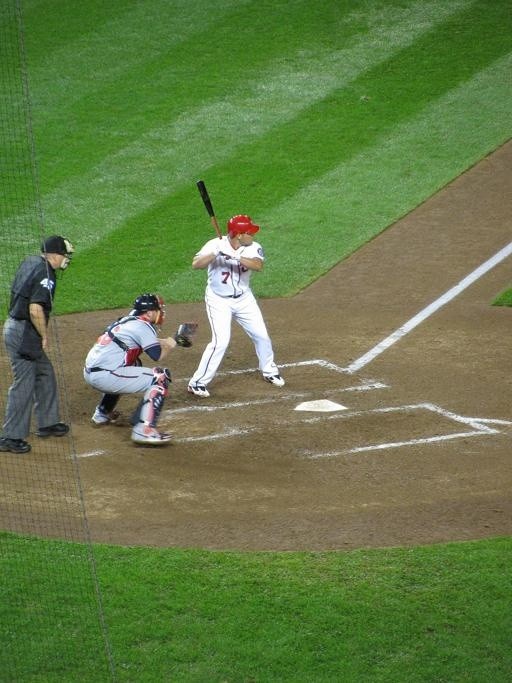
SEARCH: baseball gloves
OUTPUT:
[175,323,198,347]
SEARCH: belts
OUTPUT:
[91,367,103,372]
[230,295,241,298]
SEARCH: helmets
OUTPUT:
[228,215,259,234]
[129,294,163,315]
[42,236,75,254]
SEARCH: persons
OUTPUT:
[186,215,286,398]
[2,234,79,454]
[81,292,190,447]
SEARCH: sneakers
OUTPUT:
[132,426,171,444]
[264,375,285,386]
[37,423,69,436]
[0,438,31,453]
[188,386,210,397]
[92,405,120,424]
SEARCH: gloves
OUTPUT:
[214,238,240,261]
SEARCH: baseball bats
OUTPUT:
[197,180,231,260]
